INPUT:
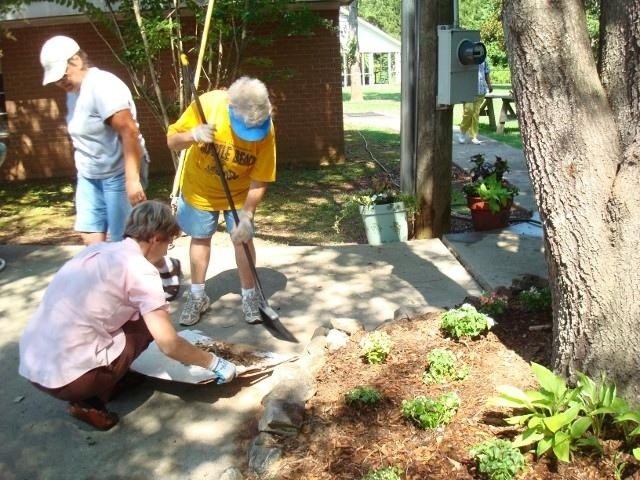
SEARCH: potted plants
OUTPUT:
[328,185,420,245]
[460,151,522,233]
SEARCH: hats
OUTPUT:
[227,102,272,142]
[39,36,80,87]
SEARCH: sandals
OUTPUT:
[159,258,181,301]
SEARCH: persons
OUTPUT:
[40,36,181,302]
[17,199,238,431]
[458,61,493,144]
[166,75,276,326]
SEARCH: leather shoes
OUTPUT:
[68,402,119,430]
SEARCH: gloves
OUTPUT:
[207,351,238,385]
[232,210,253,244]
[193,124,217,145]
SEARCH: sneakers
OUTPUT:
[242,292,264,324]
[178,292,209,326]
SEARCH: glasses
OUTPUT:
[168,239,175,250]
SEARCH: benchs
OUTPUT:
[479,94,521,137]
